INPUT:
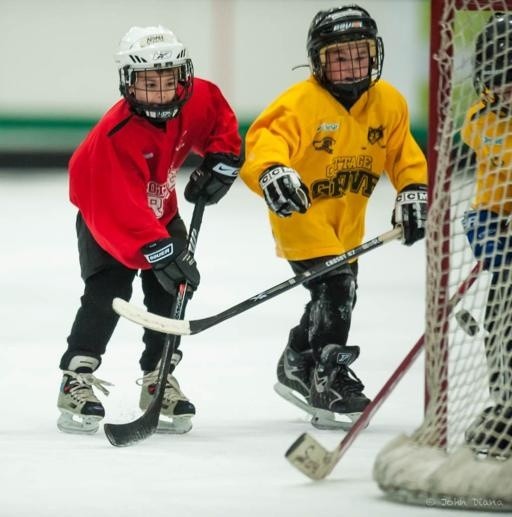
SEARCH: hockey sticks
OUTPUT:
[103,197,205,448]
[112,226,403,336]
[284,262,482,482]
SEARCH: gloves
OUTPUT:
[459,209,512,273]
[138,236,201,301]
[258,163,313,219]
[185,152,245,208]
[392,182,430,248]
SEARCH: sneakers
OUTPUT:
[465,404,511,457]
[56,351,115,417]
[309,345,372,415]
[136,350,197,419]
[277,325,316,400]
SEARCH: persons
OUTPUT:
[241,4,427,431]
[459,13,512,459]
[57,25,241,435]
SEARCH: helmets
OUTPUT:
[471,11,512,96]
[305,3,386,102]
[116,23,195,125]
